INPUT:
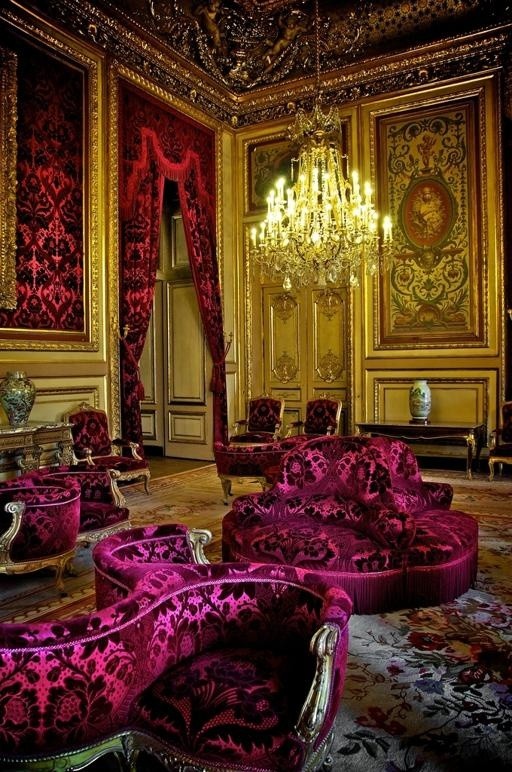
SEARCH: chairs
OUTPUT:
[209,393,343,506]
[60,401,155,506]
[486,400,511,483]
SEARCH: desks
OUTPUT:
[353,418,485,481]
[0,418,75,480]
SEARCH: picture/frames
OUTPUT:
[355,72,501,365]
[1,7,104,360]
[234,108,359,218]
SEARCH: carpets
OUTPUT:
[0,459,510,772]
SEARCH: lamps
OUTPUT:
[241,1,396,298]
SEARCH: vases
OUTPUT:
[0,367,40,428]
[407,378,432,420]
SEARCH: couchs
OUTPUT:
[0,461,136,597]
[1,521,359,771]
[219,430,480,620]
